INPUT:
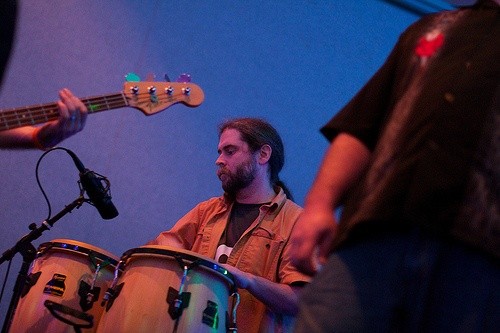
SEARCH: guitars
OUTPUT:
[0,73,206,131]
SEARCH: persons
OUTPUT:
[284,0,499,332]
[146,118,321,333]
[0,1,89,152]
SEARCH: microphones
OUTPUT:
[79,168,119,220]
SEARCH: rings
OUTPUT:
[70,115,77,121]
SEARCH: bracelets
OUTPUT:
[32,125,56,151]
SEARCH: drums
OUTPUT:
[96,245,236,333]
[6,238,124,333]
